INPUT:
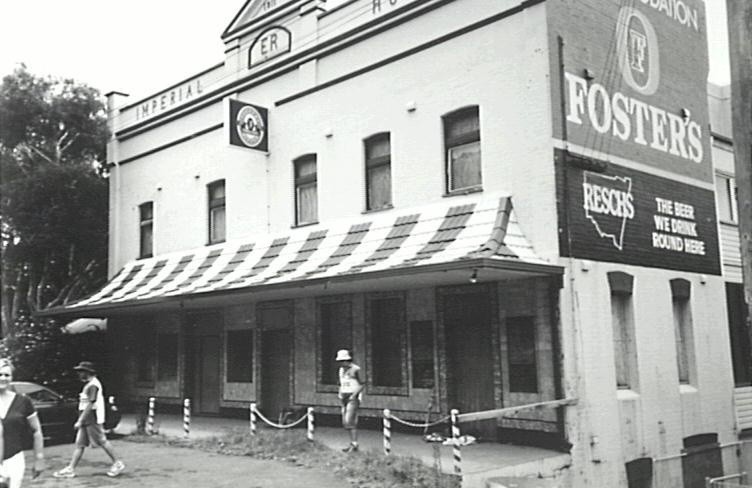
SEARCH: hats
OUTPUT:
[335,350,352,361]
[72,361,97,373]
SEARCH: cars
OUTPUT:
[8,379,124,446]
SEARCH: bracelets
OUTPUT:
[34,452,45,460]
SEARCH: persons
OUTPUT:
[334,349,367,454]
[51,360,126,479]
[0,358,47,488]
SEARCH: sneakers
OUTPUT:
[106,461,126,478]
[341,443,359,453]
[53,467,76,479]
